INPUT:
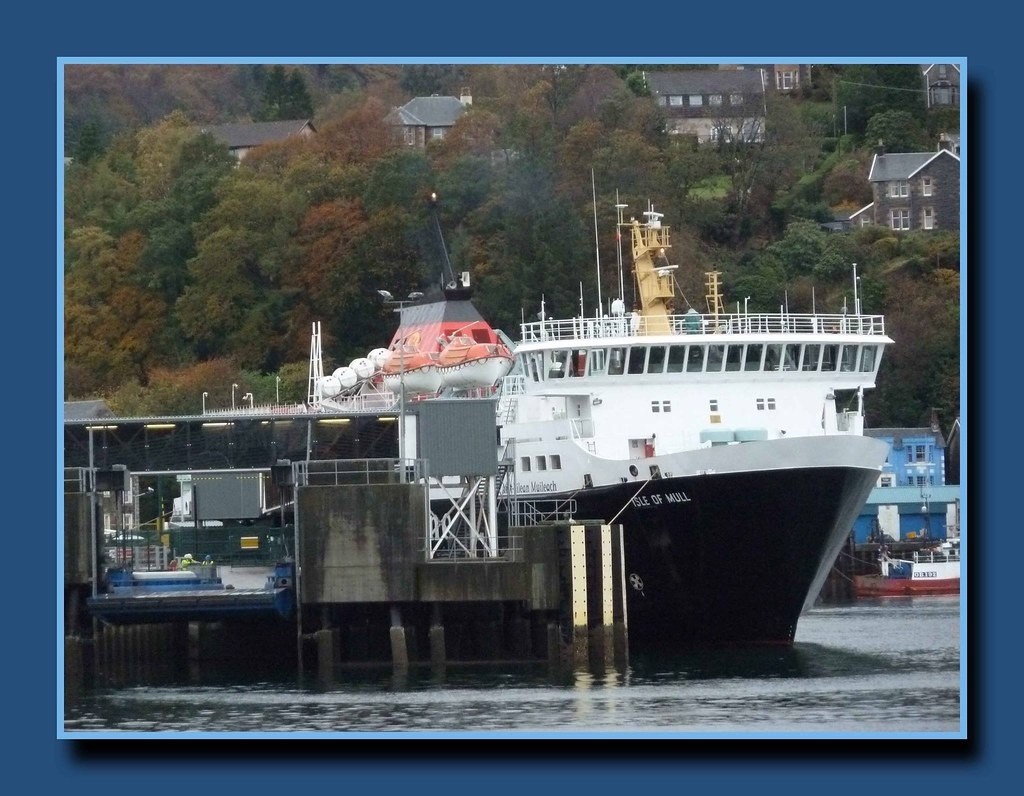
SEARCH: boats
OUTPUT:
[97,165,960,649]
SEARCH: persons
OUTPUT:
[182,554,213,571]
[686,309,701,334]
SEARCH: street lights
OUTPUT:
[203,391,208,416]
[231,383,238,412]
[276,375,281,406]
[243,392,254,410]
